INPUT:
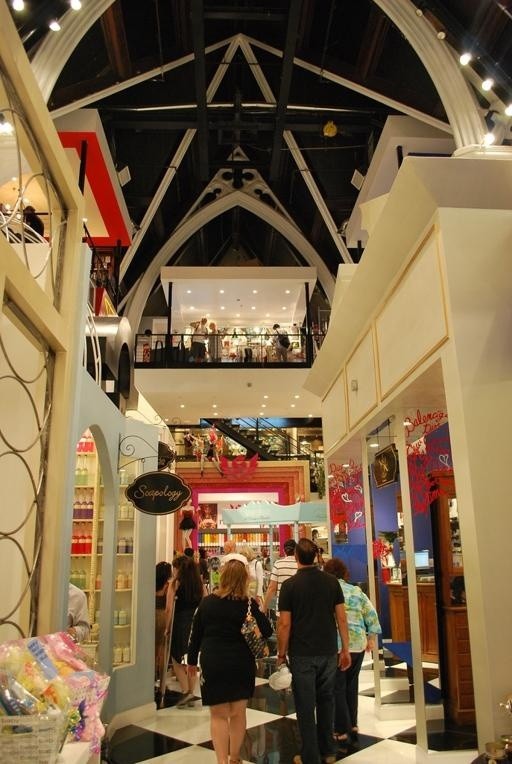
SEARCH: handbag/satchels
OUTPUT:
[240,597,269,659]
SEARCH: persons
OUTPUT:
[141,316,292,364]
[220,541,249,566]
[172,558,184,579]
[184,424,225,478]
[155,561,170,695]
[178,497,201,552]
[207,557,224,594]
[168,559,208,709]
[260,539,299,628]
[14,205,43,243]
[67,582,92,643]
[323,558,383,745]
[277,538,352,764]
[198,503,217,529]
[185,549,194,560]
[312,530,322,548]
[187,553,274,764]
[240,544,265,604]
[200,550,210,585]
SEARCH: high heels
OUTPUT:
[175,692,203,711]
[349,728,361,748]
[333,734,349,754]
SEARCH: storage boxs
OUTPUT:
[2,713,72,764]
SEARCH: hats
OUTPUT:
[269,664,292,691]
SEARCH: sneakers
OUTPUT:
[293,755,302,764]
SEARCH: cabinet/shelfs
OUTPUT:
[227,529,273,562]
[72,427,136,666]
[199,529,227,564]
[274,529,311,562]
